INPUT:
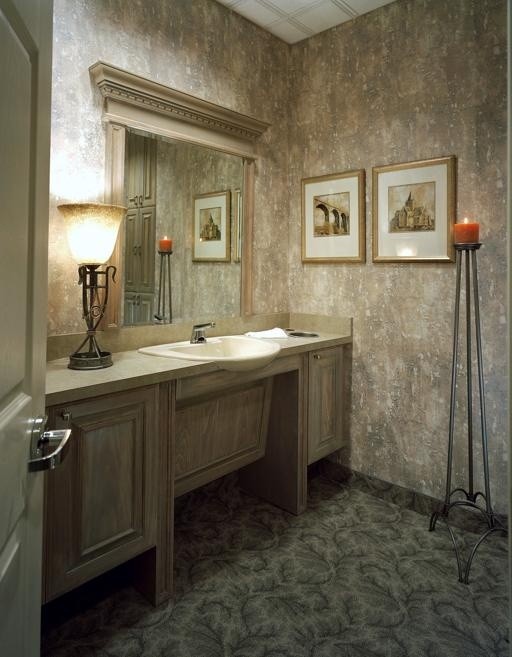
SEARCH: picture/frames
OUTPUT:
[191,189,241,263]
[300,156,457,265]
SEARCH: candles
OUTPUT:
[452,216,480,244]
[159,234,172,252]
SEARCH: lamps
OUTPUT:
[58,202,127,372]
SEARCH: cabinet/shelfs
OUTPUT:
[306,344,344,465]
[43,380,159,607]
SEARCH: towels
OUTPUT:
[246,327,288,340]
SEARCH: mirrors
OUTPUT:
[89,62,272,329]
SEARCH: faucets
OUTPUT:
[190,322,216,344]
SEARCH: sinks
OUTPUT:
[138,336,282,373]
